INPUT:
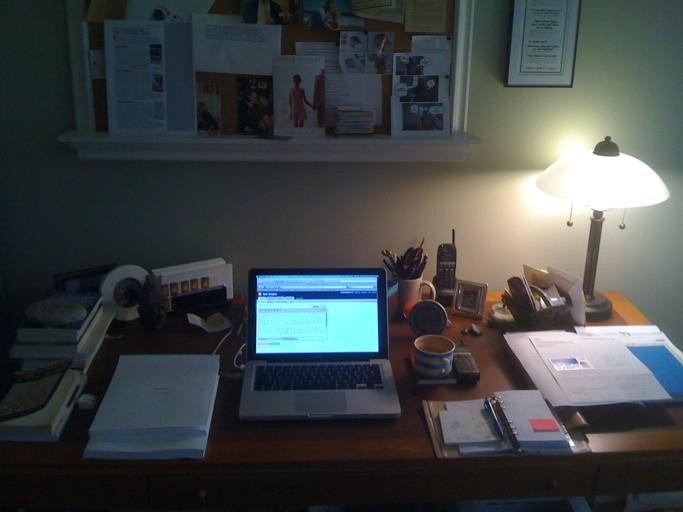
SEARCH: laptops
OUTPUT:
[236,266,401,420]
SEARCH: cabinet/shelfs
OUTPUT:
[57,0,477,179]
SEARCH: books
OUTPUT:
[1,291,221,459]
[422,389,592,459]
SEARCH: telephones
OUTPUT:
[432,229,456,307]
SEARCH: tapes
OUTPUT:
[98,262,156,323]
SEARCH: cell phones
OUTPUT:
[452,335,481,386]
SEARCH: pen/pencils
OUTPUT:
[381,237,427,278]
[483,397,505,441]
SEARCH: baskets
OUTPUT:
[509,286,572,326]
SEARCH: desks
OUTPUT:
[0,291,683,512]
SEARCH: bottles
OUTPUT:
[397,274,436,313]
[414,334,456,377]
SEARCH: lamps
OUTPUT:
[536,136,670,322]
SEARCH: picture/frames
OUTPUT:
[451,280,488,320]
[507,0,581,88]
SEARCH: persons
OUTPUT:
[313,69,325,128]
[290,75,316,128]
[237,91,272,134]
[311,0,340,32]
[345,34,392,76]
[198,103,219,135]
[117,280,142,308]
